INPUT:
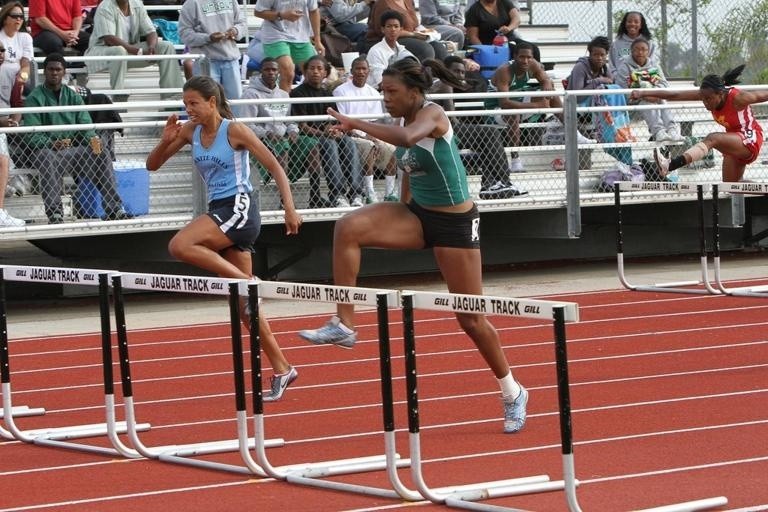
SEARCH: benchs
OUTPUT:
[0,5,764,184]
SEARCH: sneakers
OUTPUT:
[669,133,684,141]
[656,129,671,142]
[479,180,513,199]
[298,316,356,350]
[350,196,364,207]
[366,196,378,204]
[244,275,263,316]
[550,159,565,170]
[384,191,399,202]
[507,185,528,197]
[0,175,133,226]
[503,381,528,433]
[654,146,671,177]
[335,195,351,208]
[262,364,298,402]
[510,162,527,173]
[576,132,597,144]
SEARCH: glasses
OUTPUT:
[8,12,24,19]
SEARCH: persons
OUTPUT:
[627,65,768,195]
[146,75,303,401]
[298,59,529,434]
[0,0,686,227]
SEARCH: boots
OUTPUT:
[279,200,284,210]
[309,172,331,208]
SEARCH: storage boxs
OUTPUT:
[73,160,150,217]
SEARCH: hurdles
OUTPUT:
[400,288,728,511]
[0,266,255,460]
[111,270,400,477]
[709,184,767,297]
[615,179,767,297]
[242,278,551,500]
